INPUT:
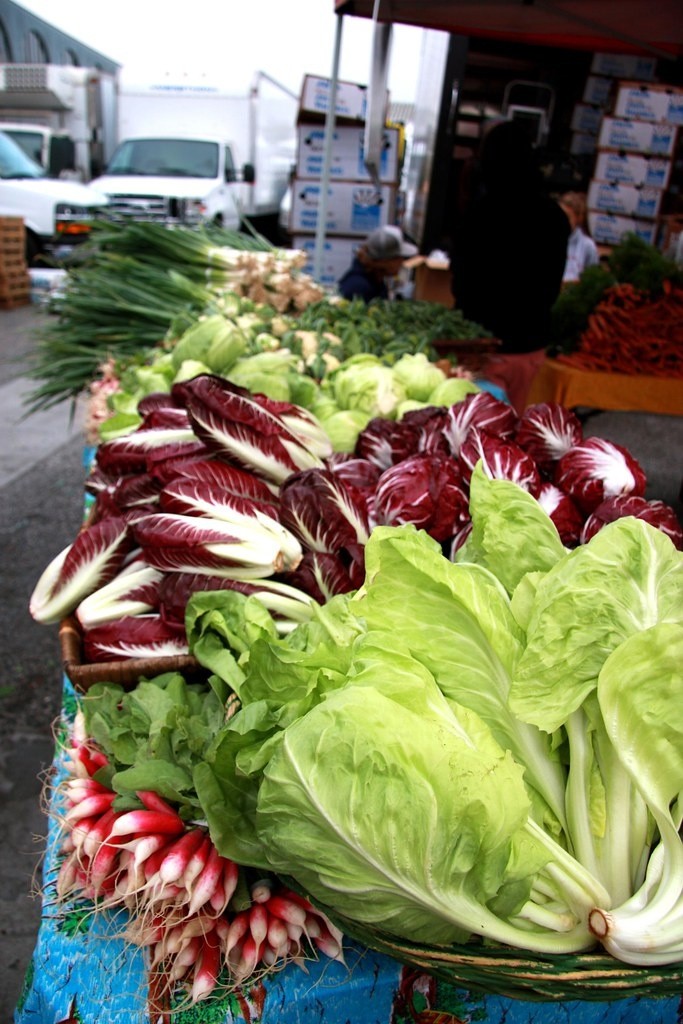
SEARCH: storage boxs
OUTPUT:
[293,73,457,309]
[568,51,682,251]
[0,214,30,310]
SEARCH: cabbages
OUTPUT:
[100,313,480,453]
[27,371,683,663]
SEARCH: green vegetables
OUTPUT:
[298,296,490,358]
[174,456,683,968]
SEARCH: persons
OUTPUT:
[560,192,599,289]
[450,122,570,335]
[339,227,419,304]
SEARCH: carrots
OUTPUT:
[556,280,683,378]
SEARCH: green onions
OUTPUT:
[13,199,324,421]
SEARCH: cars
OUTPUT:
[0,123,79,180]
[1,126,112,268]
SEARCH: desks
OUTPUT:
[524,362,683,414]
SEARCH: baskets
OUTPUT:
[60,502,209,690]
[226,691,683,1002]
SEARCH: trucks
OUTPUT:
[85,90,289,240]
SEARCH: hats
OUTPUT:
[366,225,417,260]
